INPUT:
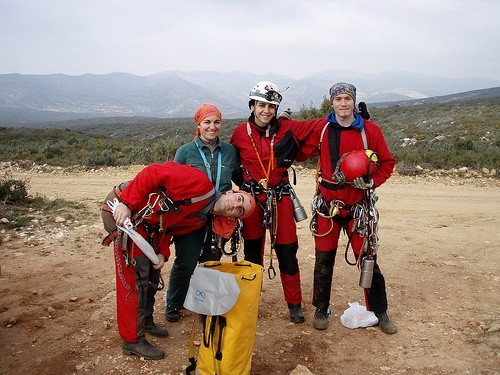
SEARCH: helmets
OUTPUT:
[248,80,282,106]
[340,147,380,183]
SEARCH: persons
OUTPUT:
[227,81,370,324]
[166,104,243,322]
[279,81,397,334]
[102,162,255,361]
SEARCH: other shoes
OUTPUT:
[313,308,331,329]
[374,309,397,335]
[287,302,306,323]
[166,308,180,322]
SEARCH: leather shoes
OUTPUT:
[146,323,170,339]
[123,340,164,359]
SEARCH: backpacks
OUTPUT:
[182,261,263,375]
